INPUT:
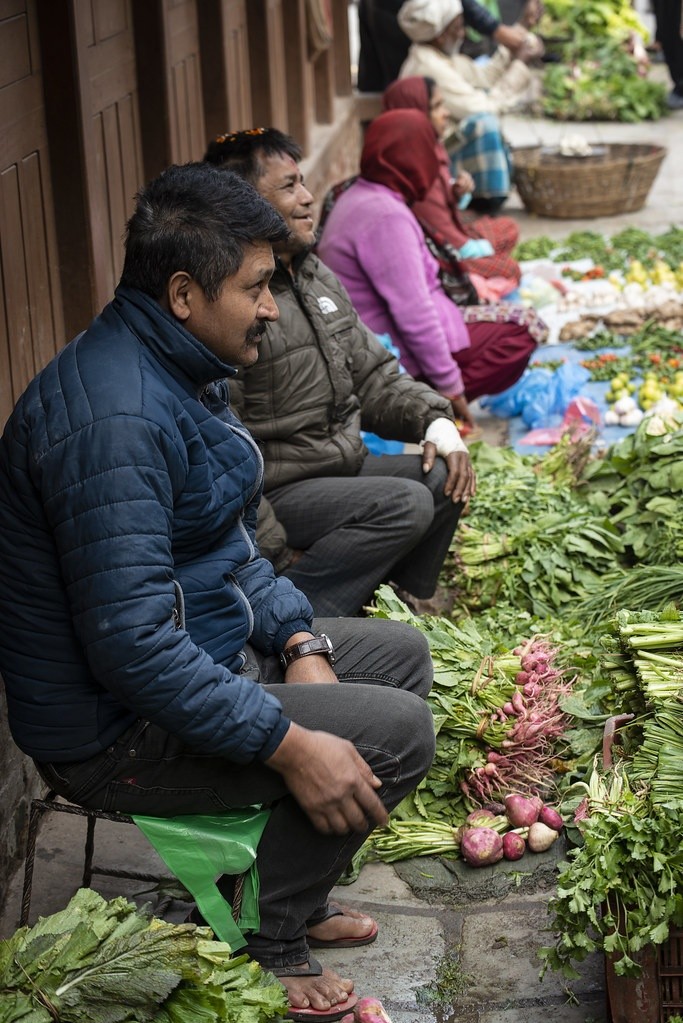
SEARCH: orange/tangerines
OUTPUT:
[604,373,683,408]
[611,257,683,295]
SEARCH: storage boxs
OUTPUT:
[599,890,683,1023]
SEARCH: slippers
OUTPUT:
[301,901,379,950]
[181,916,360,1023]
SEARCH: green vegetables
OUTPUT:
[0,887,290,1023]
[441,409,683,982]
[528,0,671,123]
[508,223,683,270]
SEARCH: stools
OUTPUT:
[19,790,274,960]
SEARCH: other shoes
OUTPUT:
[664,92,683,111]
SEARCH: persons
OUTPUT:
[0,171,433,1023]
[204,125,474,617]
[399,1,546,209]
[648,1,683,109]
[386,76,521,301]
[313,108,549,424]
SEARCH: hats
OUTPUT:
[395,0,464,43]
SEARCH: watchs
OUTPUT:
[280,633,336,667]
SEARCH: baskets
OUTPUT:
[503,141,669,219]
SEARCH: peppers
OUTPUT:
[573,314,683,382]
